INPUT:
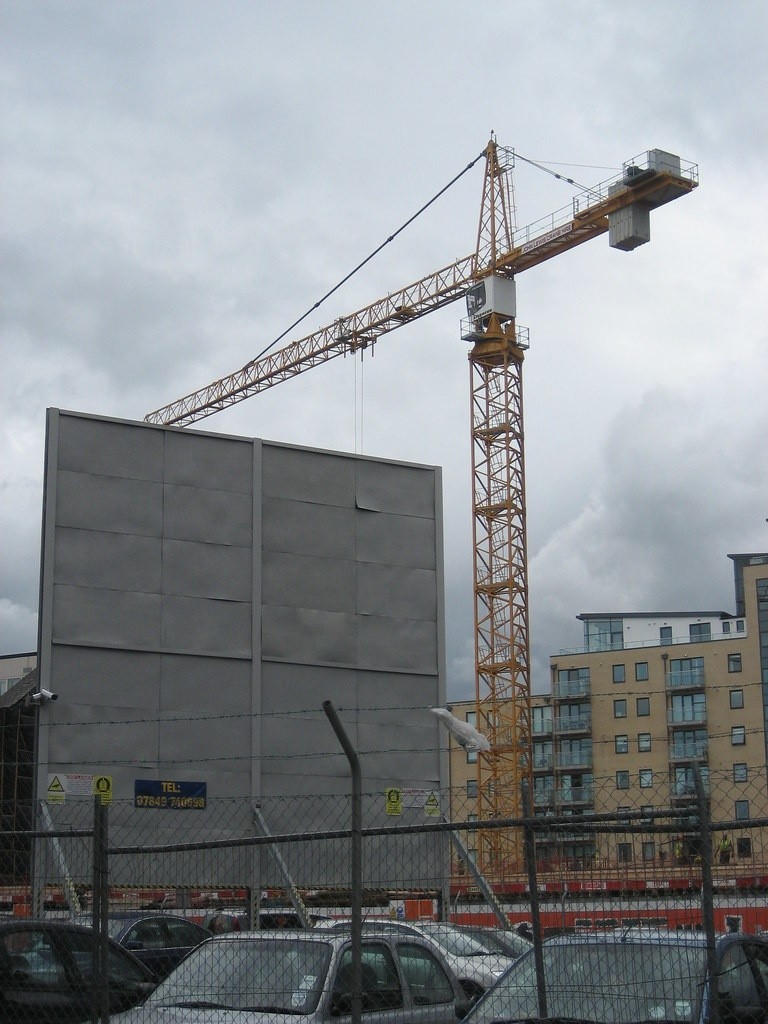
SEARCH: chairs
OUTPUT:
[136,927,162,941]
[334,962,377,992]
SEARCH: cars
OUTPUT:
[18,910,218,994]
[282,914,536,1020]
[458,926,768,1024]
[1,914,163,1024]
[81,926,471,1024]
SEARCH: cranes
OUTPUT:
[142,128,704,881]
